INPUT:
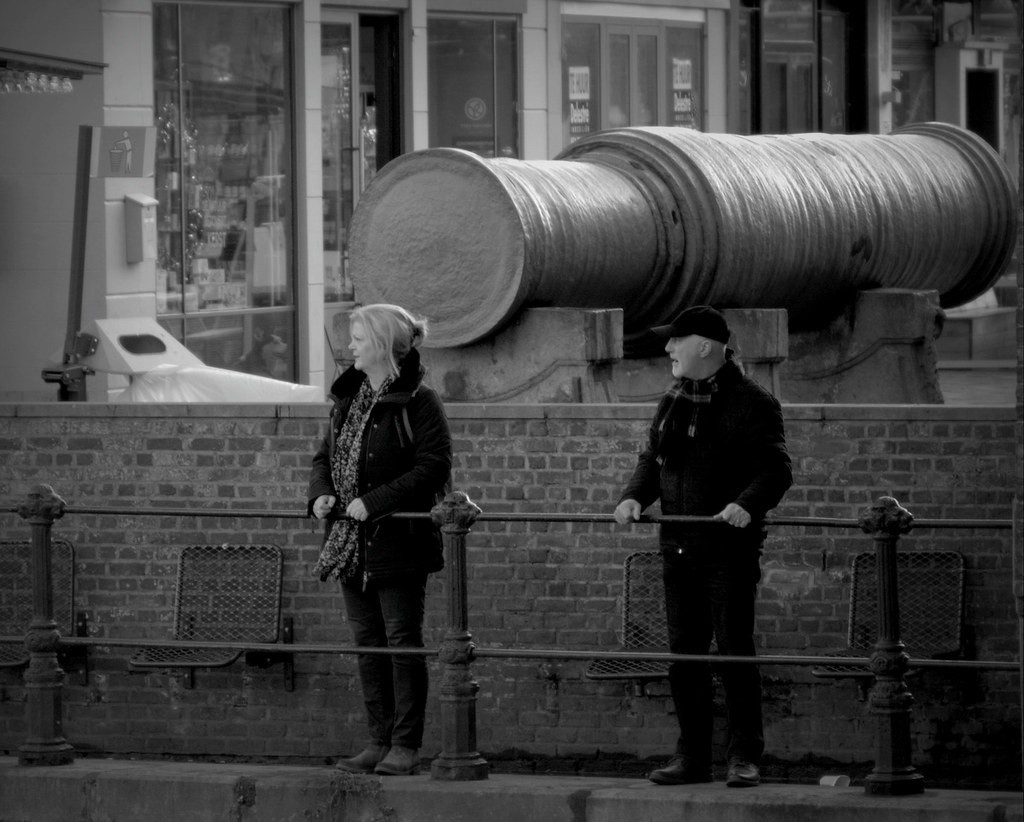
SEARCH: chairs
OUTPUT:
[813,551,970,702]
[0,538,88,686]
[131,544,295,694]
[583,550,675,695]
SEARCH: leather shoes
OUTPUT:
[649,754,713,785]
[726,756,760,787]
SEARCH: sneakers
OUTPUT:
[336,744,421,776]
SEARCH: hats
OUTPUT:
[650,306,731,344]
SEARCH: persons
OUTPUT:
[302,302,453,777]
[612,302,792,789]
